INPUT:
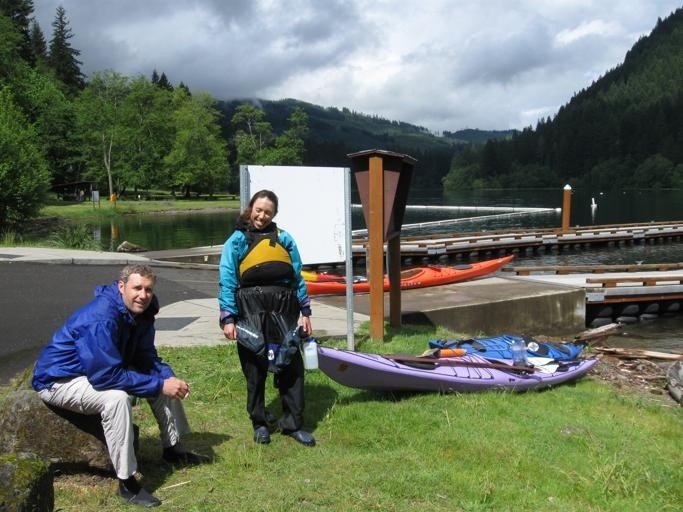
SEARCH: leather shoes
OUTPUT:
[252,424,272,445]
[280,427,316,448]
[162,447,212,467]
[115,479,162,509]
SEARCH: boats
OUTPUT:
[317,347,597,395]
[300,254,515,296]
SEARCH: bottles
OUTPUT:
[235,321,264,348]
[512,337,528,367]
[303,338,319,369]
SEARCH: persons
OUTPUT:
[31,265,212,508]
[218,190,316,445]
[110,218,118,245]
[110,192,116,210]
[78,188,85,203]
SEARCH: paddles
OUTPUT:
[382,355,543,371]
